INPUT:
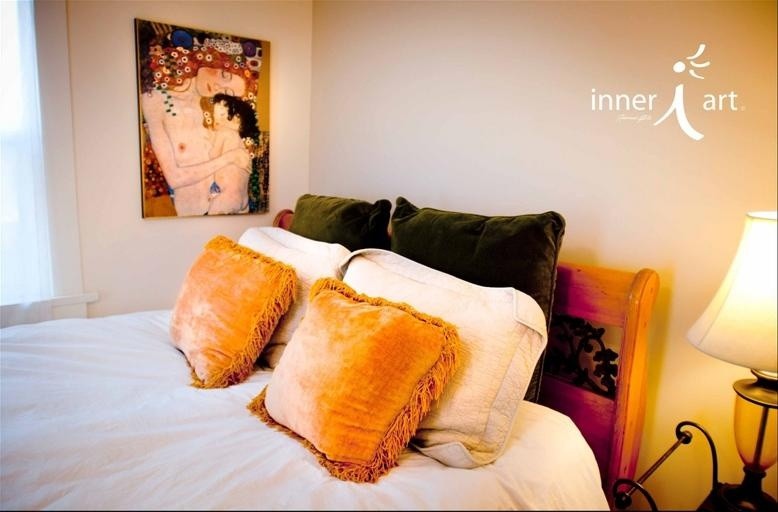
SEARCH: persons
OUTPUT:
[140,37,258,220]
[204,91,261,215]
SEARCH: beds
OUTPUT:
[2,207,660,511]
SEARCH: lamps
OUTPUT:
[685,207,778,511]
